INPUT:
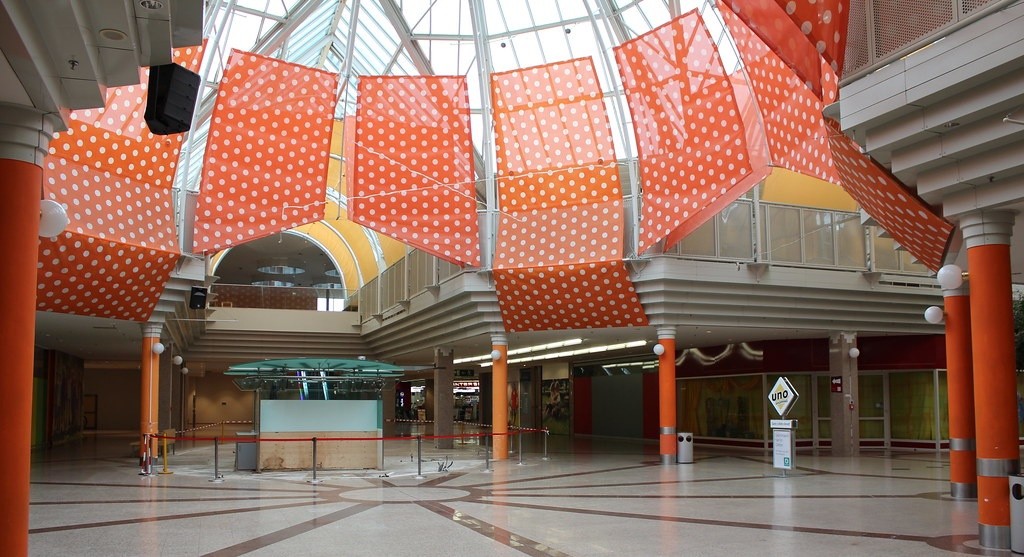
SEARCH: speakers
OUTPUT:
[144,63,201,136]
[188,286,208,310]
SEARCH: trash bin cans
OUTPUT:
[235,432,257,471]
[1008,472,1024,555]
[677,432,694,463]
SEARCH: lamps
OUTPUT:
[653,344,666,356]
[924,306,948,322]
[454,337,647,367]
[848,348,859,359]
[151,342,188,374]
[37,200,69,238]
[937,263,969,289]
